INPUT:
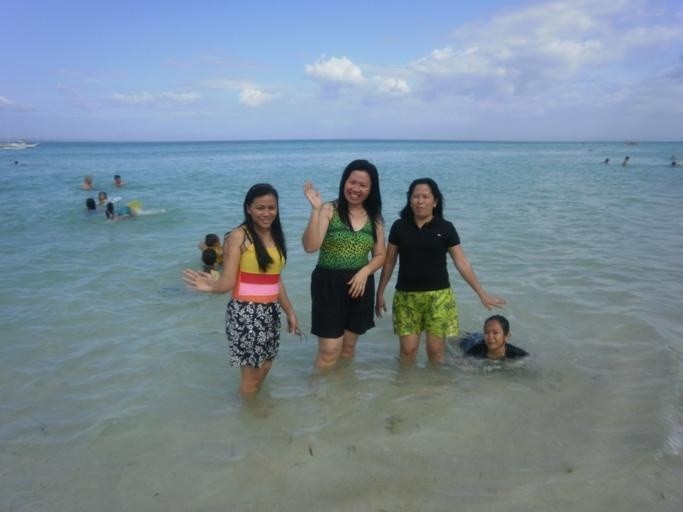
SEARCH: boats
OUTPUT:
[2,142,37,150]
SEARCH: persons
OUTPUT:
[98,192,106,204]
[197,233,222,255]
[104,202,136,221]
[600,158,610,165]
[374,178,509,369]
[456,313,528,360]
[301,158,388,372]
[198,248,221,280]
[114,175,124,186]
[669,161,682,168]
[85,198,96,209]
[182,183,296,400]
[81,175,92,189]
[622,156,629,165]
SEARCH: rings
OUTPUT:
[496,299,497,302]
